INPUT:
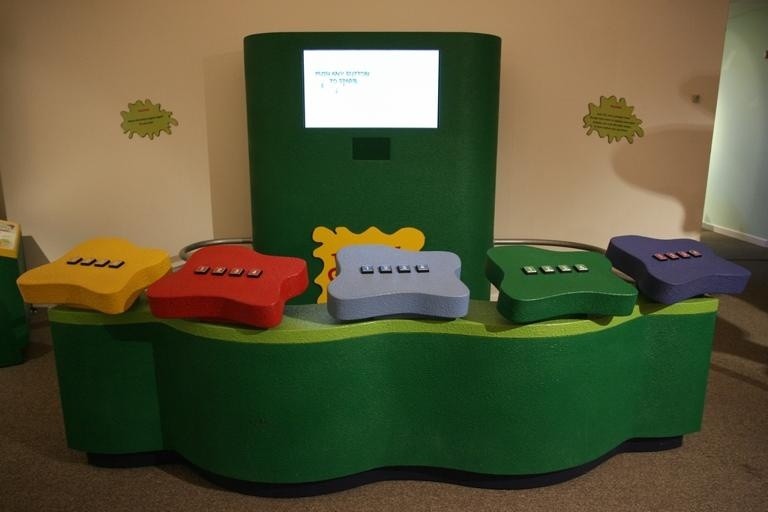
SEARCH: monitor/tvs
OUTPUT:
[303,49,439,129]
